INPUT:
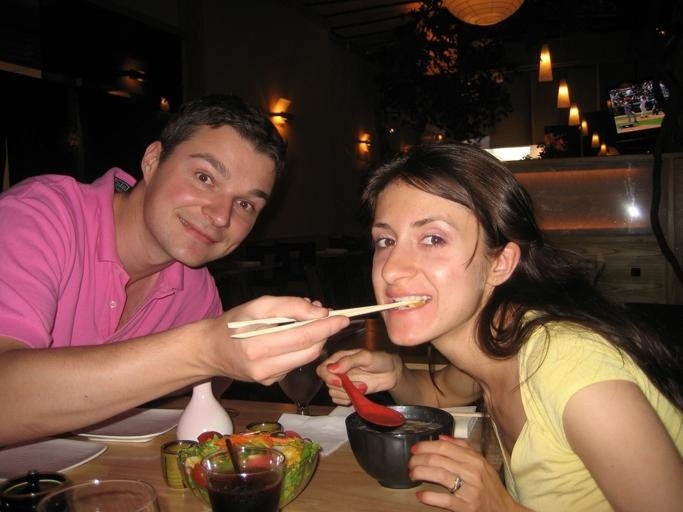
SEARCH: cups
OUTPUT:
[34,477,160,512]
[199,445,285,512]
[159,439,198,489]
[245,420,283,433]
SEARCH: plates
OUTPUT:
[0,437,108,483]
[71,407,184,444]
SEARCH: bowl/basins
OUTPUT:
[344,403,456,490]
[176,430,320,512]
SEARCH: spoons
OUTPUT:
[335,370,406,429]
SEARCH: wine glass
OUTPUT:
[277,349,329,417]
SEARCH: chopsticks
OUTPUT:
[449,412,490,418]
[225,299,420,340]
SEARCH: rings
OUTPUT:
[449,475,464,493]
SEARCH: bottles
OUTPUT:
[176,376,234,442]
[0,470,74,512]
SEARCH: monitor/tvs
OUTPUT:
[605,77,672,136]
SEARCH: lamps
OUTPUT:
[442,0,524,26]
[538,44,610,157]
[266,97,294,126]
[357,132,373,154]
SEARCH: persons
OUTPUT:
[314,140,683,511]
[0,94,351,449]
[610,80,668,123]
